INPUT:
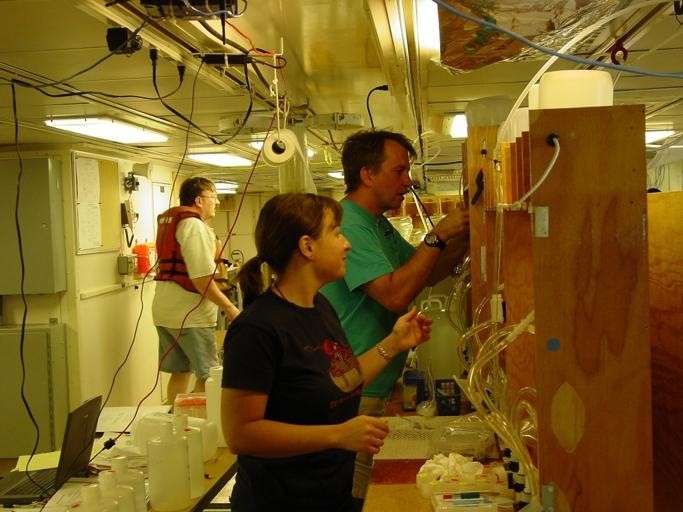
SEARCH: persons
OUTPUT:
[221,190,433,512]
[313,129,470,512]
[150,176,240,413]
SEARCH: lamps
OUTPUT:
[185,144,254,168]
[645,121,675,144]
[213,178,239,195]
[43,103,169,145]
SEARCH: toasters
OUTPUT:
[77,454,147,512]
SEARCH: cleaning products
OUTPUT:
[70,365,225,512]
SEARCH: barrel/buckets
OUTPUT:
[416,306,467,395]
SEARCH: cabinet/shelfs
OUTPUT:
[358,102,656,512]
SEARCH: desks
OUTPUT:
[0,431,239,512]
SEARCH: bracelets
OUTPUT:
[375,344,390,362]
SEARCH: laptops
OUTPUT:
[1,394,104,502]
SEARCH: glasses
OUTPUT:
[200,194,221,202]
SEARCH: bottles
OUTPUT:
[203,365,227,448]
[147,421,190,512]
[172,414,205,499]
[131,412,217,464]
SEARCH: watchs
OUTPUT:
[423,232,445,250]
